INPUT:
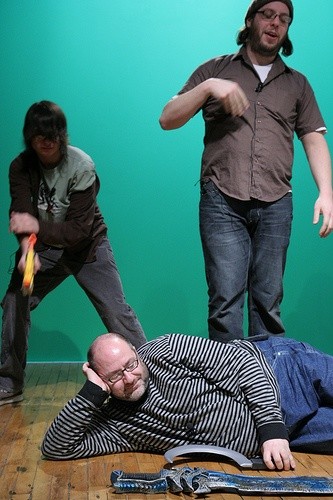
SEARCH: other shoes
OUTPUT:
[0,391,24,407]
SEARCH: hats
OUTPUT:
[244,0,294,25]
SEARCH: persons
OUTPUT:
[158,0,333,344]
[40,333,333,471]
[0,100,147,405]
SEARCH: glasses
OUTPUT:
[254,7,291,24]
[99,347,139,384]
